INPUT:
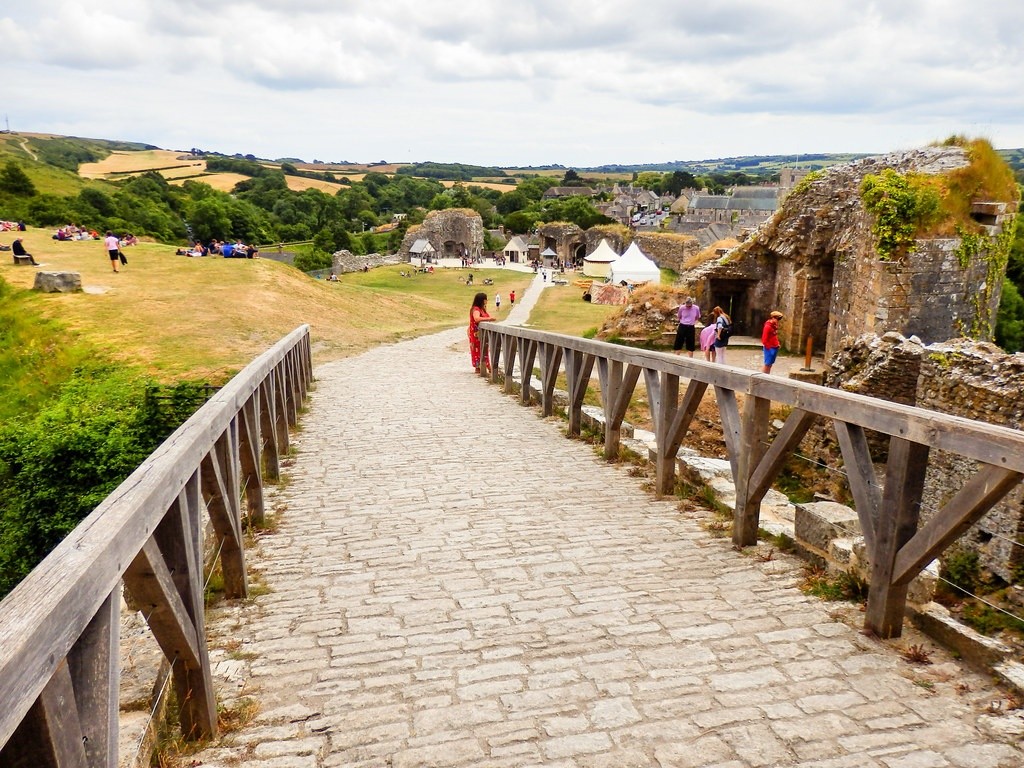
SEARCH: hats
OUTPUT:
[770,311,783,318]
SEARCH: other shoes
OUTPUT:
[113,269,120,273]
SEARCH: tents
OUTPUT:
[609,240,660,286]
[583,239,620,277]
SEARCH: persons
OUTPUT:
[762,312,783,375]
[12,237,40,265]
[0,219,26,231]
[118,232,139,247]
[178,239,260,259]
[104,230,122,273]
[57,222,99,241]
[700,307,731,364]
[467,292,496,374]
[491,251,507,267]
[330,272,342,283]
[0,243,11,252]
[399,265,434,278]
[532,258,581,283]
[495,291,500,311]
[675,297,700,358]
[510,291,515,308]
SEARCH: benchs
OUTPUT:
[13,254,31,265]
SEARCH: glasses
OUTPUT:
[685,304,692,307]
[777,317,782,320]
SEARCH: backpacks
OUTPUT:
[720,317,732,337]
